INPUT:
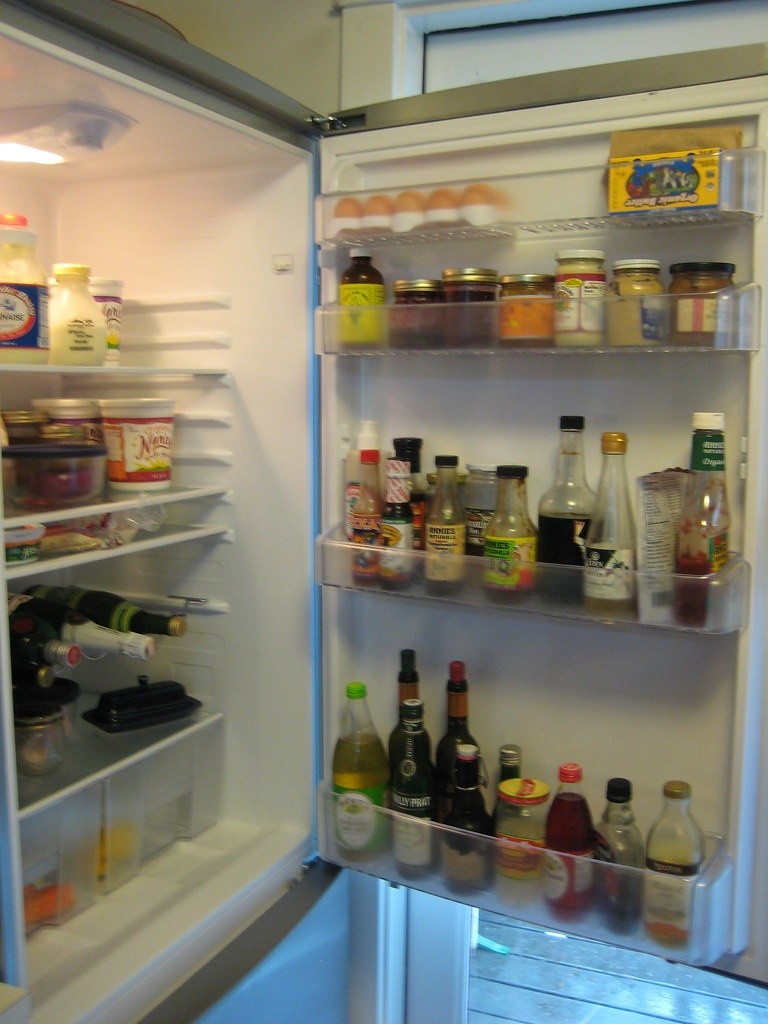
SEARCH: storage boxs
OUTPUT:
[608,148,725,216]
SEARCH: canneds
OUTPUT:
[0,226,51,367]
[423,462,509,578]
[2,410,52,495]
[493,778,554,903]
[388,248,739,349]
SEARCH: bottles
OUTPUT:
[12,704,66,777]
[467,462,500,556]
[334,648,706,948]
[1,398,177,510]
[442,267,497,348]
[8,609,81,667]
[14,677,81,739]
[345,450,392,542]
[393,280,443,351]
[484,465,537,603]
[424,456,467,595]
[341,247,387,349]
[22,585,186,636]
[6,592,154,660]
[381,459,414,589]
[555,245,605,345]
[497,274,554,348]
[606,259,665,348]
[536,416,597,607]
[426,472,468,503]
[666,262,736,344]
[354,451,382,583]
[676,412,731,626]
[0,212,121,366]
[585,433,638,615]
[394,438,426,581]
[8,652,54,689]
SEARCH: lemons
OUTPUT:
[68,820,140,883]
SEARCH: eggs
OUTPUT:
[335,185,508,220]
